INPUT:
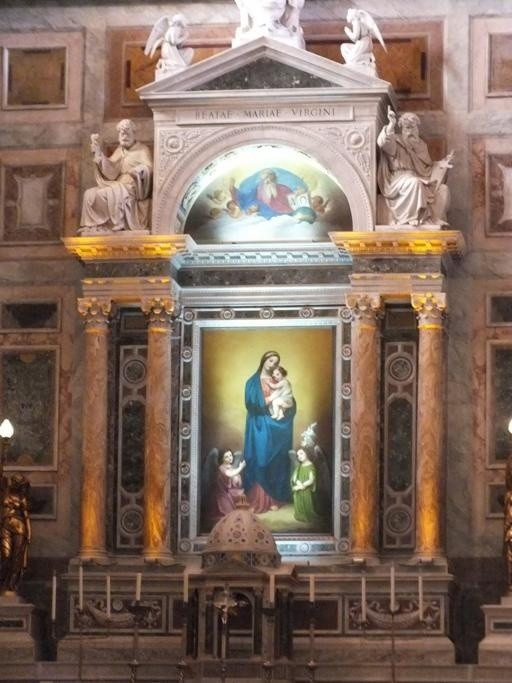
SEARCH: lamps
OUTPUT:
[0,418,14,465]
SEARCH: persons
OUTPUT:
[205,168,334,223]
[0,471,33,592]
[286,445,332,522]
[144,10,194,74]
[340,8,388,66]
[376,104,458,227]
[203,446,248,518]
[242,350,298,512]
[73,119,154,233]
[264,365,295,421]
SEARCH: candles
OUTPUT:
[183,569,188,602]
[361,576,366,622]
[390,567,395,610]
[310,574,315,602]
[418,575,423,621]
[270,574,275,602]
[52,566,142,620]
